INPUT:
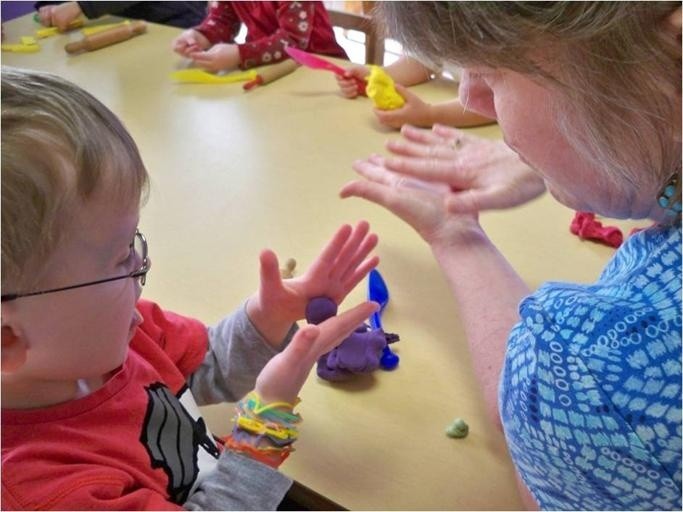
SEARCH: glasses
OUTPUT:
[2,227,151,301]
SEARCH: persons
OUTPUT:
[33,1,208,33]
[174,1,349,72]
[0,65,383,510]
[338,3,683,511]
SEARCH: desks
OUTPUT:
[1,6,640,511]
